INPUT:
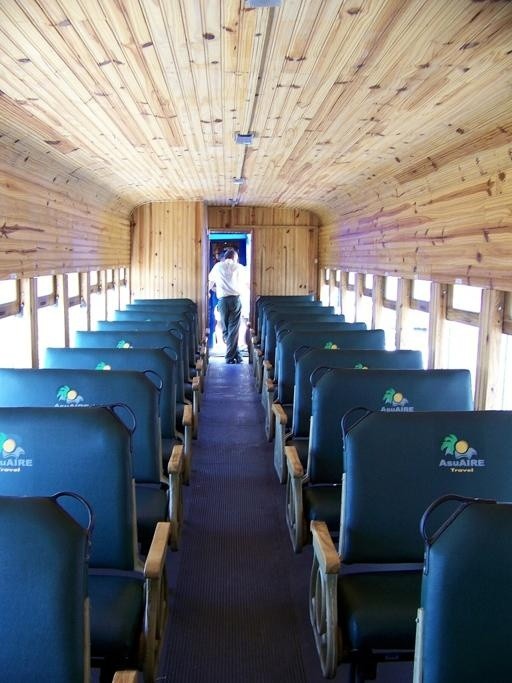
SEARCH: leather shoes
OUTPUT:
[229,360,235,364]
[237,358,243,363]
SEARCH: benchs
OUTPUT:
[0,298,210,683]
[247,295,512,683]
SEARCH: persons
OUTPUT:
[206,248,249,365]
